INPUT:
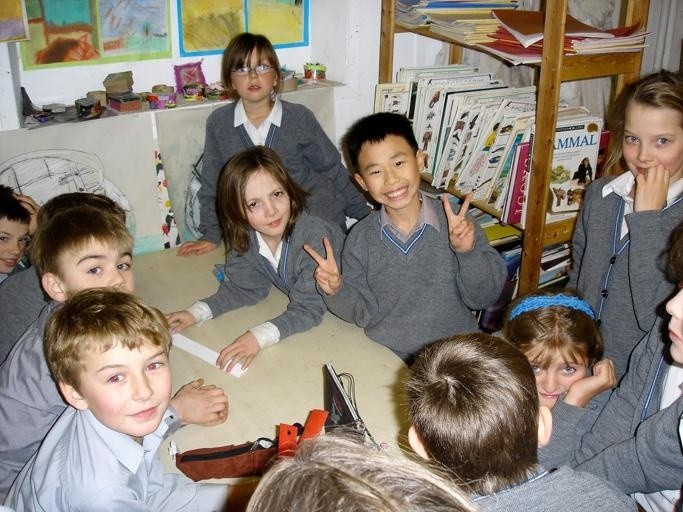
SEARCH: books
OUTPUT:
[395,1,655,66]
[108,95,141,111]
[103,70,134,94]
[375,63,603,228]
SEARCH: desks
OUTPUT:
[131,238,412,484]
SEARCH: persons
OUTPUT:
[501,68,683,511]
[175,32,373,257]
[1,184,260,512]
[246,333,638,512]
[163,145,346,373]
[302,111,509,368]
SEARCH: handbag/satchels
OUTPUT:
[176,442,277,482]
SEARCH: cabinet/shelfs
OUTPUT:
[378,1,652,299]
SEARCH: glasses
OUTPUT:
[230,64,276,75]
